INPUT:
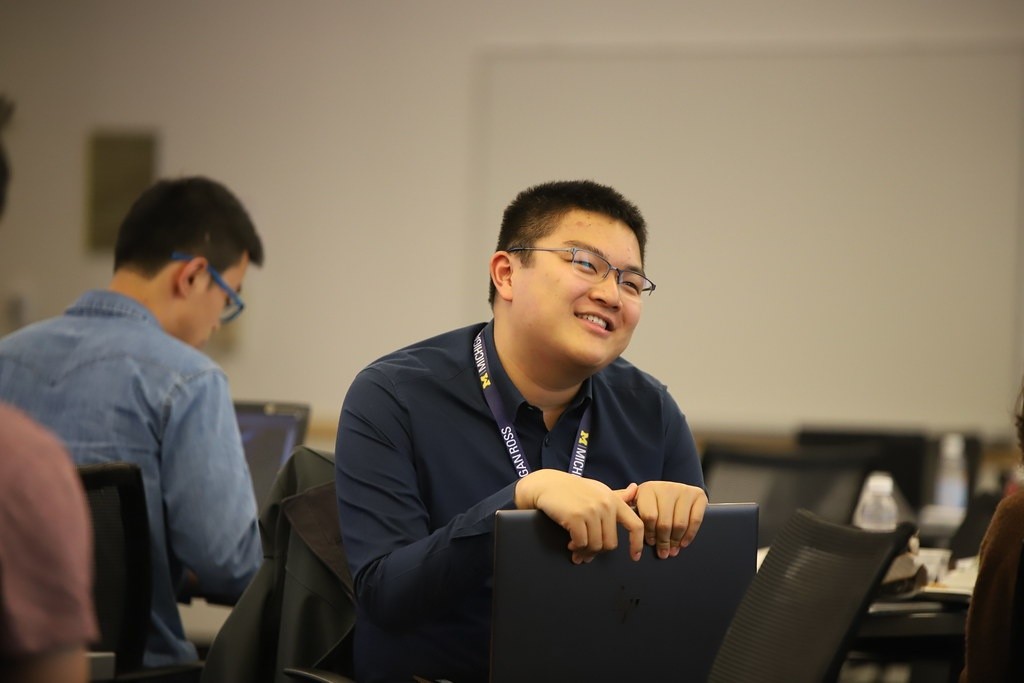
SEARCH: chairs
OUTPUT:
[278,499,760,682]
[78,461,209,683]
[703,503,919,683]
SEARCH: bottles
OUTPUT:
[931,430,969,513]
[860,475,899,536]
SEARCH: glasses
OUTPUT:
[172,251,245,324]
[508,244,657,306]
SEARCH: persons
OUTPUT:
[3,175,267,682]
[332,179,710,680]
[0,402,95,682]
